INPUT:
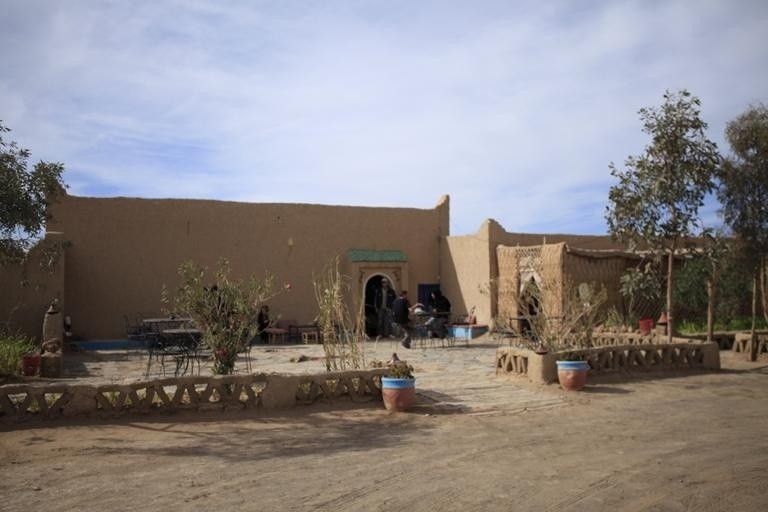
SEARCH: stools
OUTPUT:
[266,325,331,345]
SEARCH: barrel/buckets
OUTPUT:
[639,319,654,335]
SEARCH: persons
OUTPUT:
[374,278,396,341]
[419,290,452,339]
[258,305,273,344]
[391,290,420,349]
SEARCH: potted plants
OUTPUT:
[380,357,417,413]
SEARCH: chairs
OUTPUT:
[119,307,254,374]
[373,305,478,350]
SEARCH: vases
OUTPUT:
[555,358,588,391]
